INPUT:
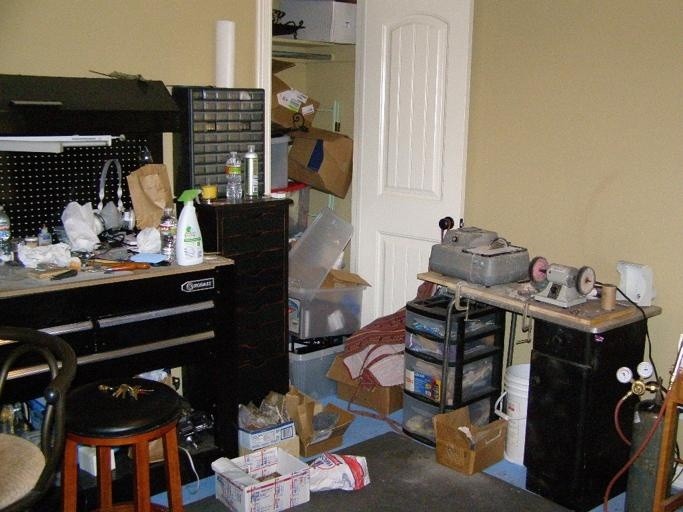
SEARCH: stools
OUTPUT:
[60,373,188,512]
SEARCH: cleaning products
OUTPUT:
[226,152,242,200]
[176,189,204,267]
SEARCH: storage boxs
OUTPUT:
[209,343,402,512]
[269,0,374,341]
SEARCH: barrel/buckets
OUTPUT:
[496,363,530,464]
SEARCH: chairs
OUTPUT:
[0,321,81,511]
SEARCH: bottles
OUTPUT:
[36,223,52,244]
[224,151,243,199]
[0,205,15,279]
[159,208,177,265]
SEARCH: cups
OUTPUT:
[24,236,38,248]
[53,226,69,243]
[200,184,218,202]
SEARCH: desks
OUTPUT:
[171,194,295,410]
[0,252,238,510]
[414,268,663,511]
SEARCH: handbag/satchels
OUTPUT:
[343,282,437,357]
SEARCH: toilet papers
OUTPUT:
[213,18,237,85]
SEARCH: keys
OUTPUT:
[98,383,155,400]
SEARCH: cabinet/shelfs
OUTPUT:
[400,294,508,478]
